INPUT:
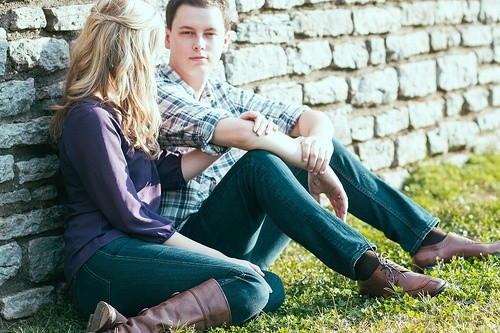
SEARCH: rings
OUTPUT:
[337,196,341,199]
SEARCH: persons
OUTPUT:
[58,0,286,333]
[156,0,499,333]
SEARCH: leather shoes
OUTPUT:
[357,237,448,299]
[413,232,500,274]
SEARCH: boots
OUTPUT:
[88,278,232,333]
